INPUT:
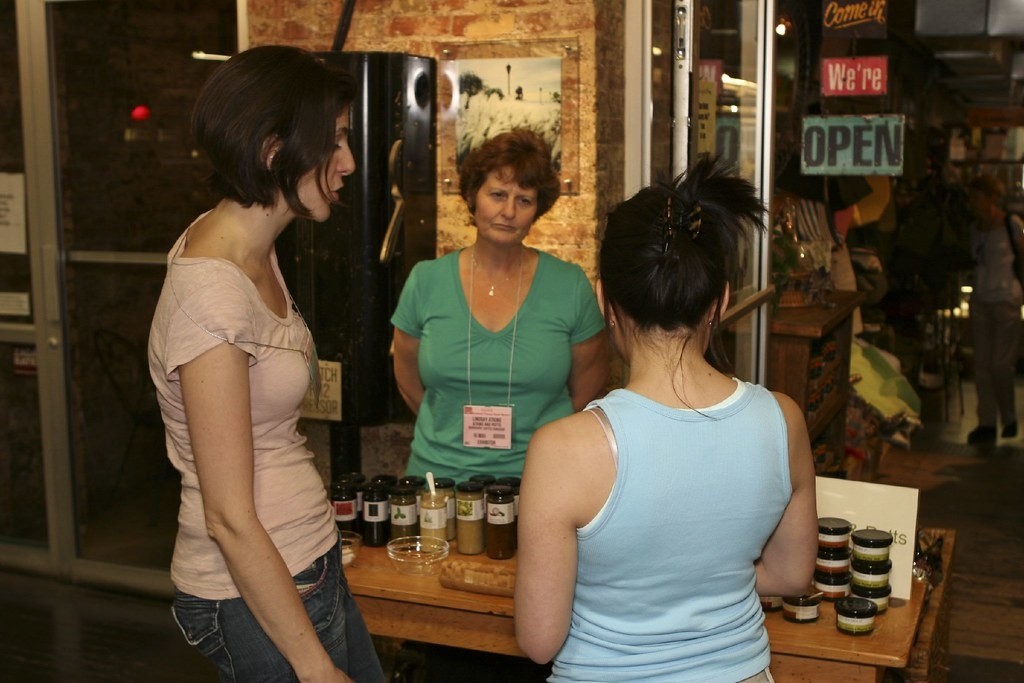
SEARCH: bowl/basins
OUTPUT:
[340,529,362,569]
[387,536,449,578]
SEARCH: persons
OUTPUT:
[390,129,607,480]
[772,126,1023,443]
[512,149,818,682]
[147,44,389,683]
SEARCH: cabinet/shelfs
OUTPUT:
[728,288,866,477]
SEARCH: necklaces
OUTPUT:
[472,247,516,297]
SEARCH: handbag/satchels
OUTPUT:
[1004,211,1024,290]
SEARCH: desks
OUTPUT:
[339,542,925,683]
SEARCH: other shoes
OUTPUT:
[969,425,996,443]
[1002,422,1017,437]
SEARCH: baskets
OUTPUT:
[772,272,834,308]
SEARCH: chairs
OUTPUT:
[90,327,164,495]
